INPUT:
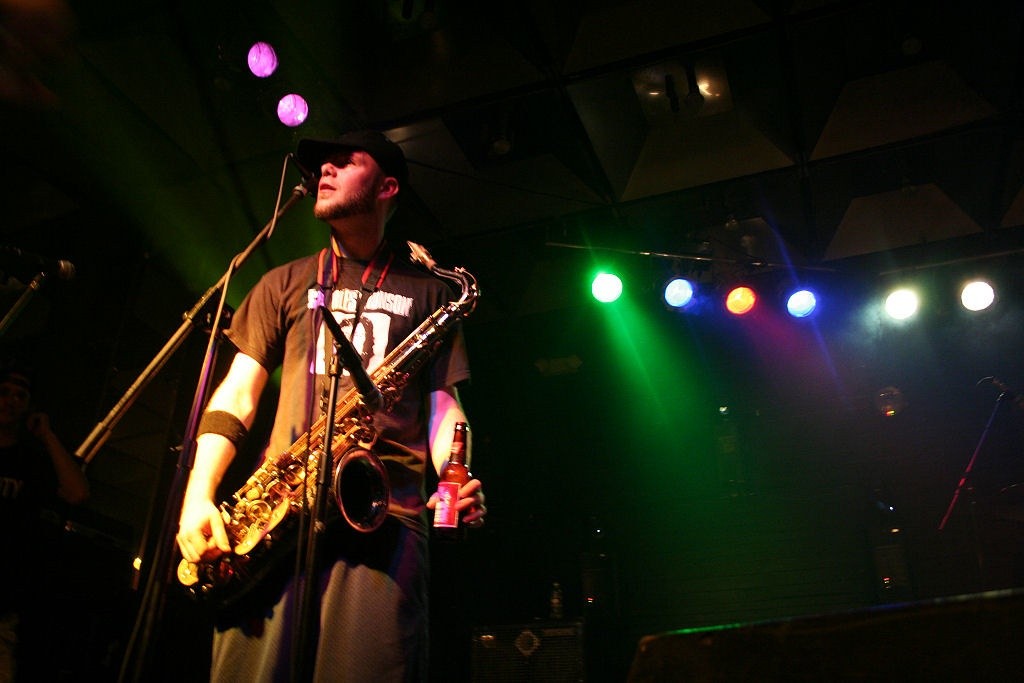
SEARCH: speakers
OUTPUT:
[624,589,1024,683]
[472,622,611,683]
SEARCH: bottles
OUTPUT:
[550,583,561,620]
[431,421,472,544]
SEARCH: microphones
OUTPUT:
[286,152,318,197]
[990,377,1024,409]
[321,307,385,411]
[0,244,75,283]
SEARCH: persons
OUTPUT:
[175,126,486,683]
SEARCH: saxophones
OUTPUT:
[175,240,484,591]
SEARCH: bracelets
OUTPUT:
[197,413,248,452]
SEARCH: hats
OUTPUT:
[296,130,410,197]
